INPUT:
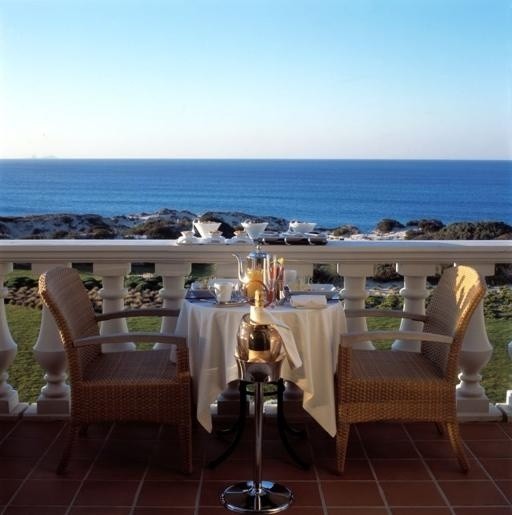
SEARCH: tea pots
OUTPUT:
[232,245,283,304]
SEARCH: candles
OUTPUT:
[254,286,264,308]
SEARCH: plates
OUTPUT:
[287,284,337,298]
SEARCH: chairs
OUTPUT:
[38,267,197,475]
[336,265,486,474]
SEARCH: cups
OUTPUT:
[193,222,222,238]
[240,222,269,240]
[208,282,232,303]
[289,222,317,234]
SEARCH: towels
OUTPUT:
[289,294,327,308]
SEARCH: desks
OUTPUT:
[219,352,295,515]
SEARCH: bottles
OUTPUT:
[247,290,271,363]
